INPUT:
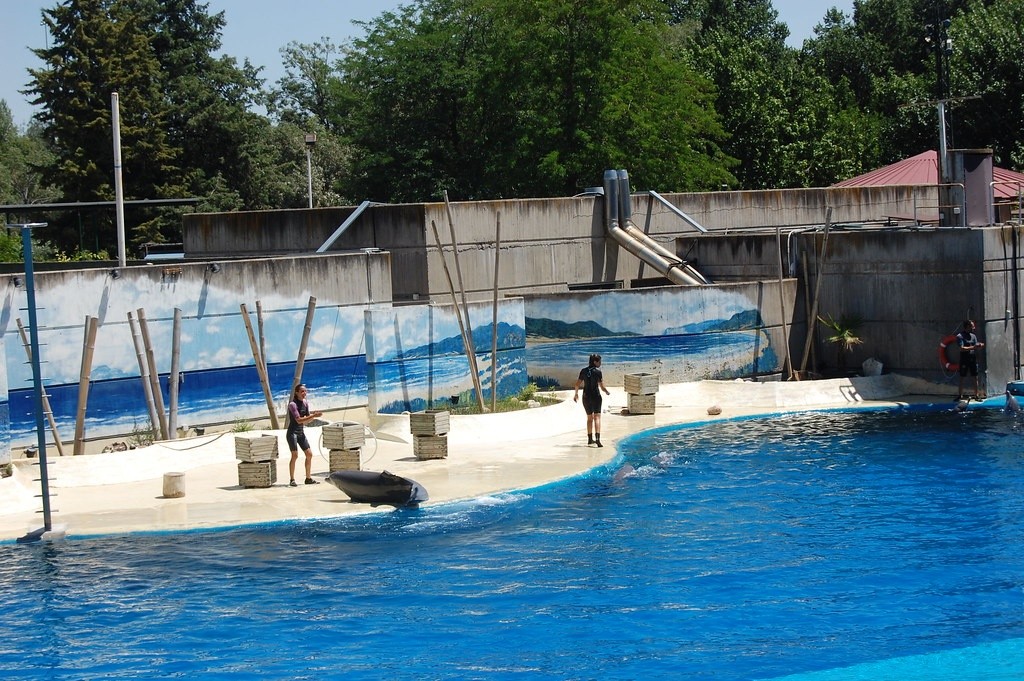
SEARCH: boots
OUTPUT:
[587,433,596,444]
[595,433,603,447]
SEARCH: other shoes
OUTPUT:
[305,479,320,484]
[954,395,963,401]
[290,479,297,486]
[975,398,982,402]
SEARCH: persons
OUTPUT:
[574,354,610,447]
[954,320,984,402]
[286,384,322,487]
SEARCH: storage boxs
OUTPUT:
[410,409,451,436]
[413,433,448,459]
[624,372,660,395]
[627,393,656,414]
[322,421,366,450]
[237,460,277,488]
[329,446,363,473]
[235,433,279,462]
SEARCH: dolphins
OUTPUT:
[325,470,429,512]
[609,462,637,491]
[948,389,1024,418]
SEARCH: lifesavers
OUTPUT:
[938,335,961,371]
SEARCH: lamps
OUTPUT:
[449,396,460,405]
[194,427,206,436]
[25,448,37,458]
